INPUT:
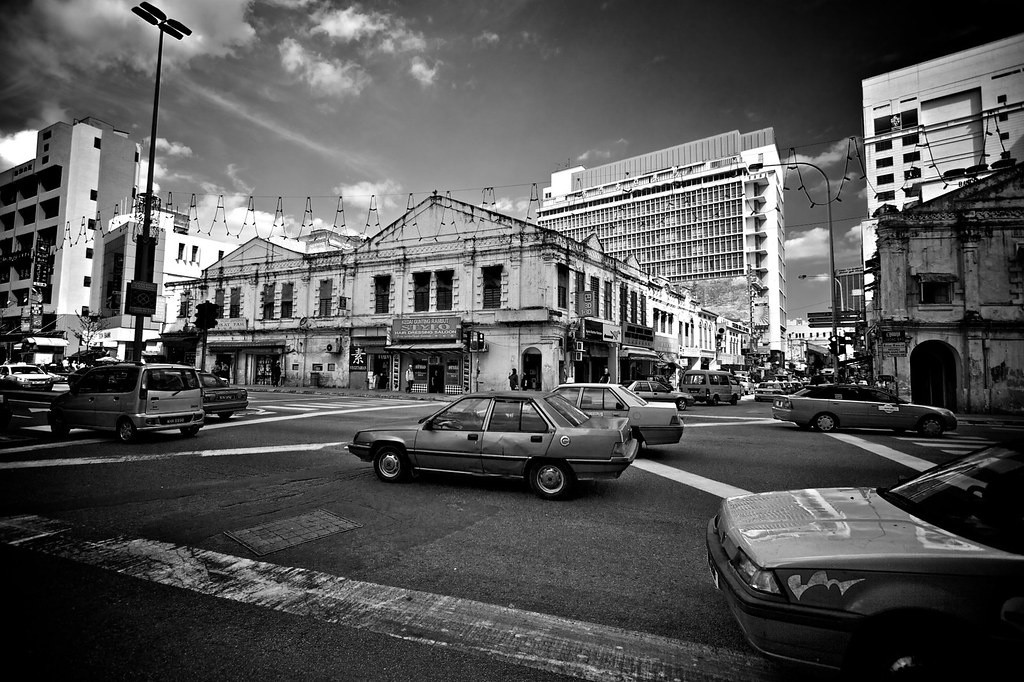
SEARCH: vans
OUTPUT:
[679,370,741,406]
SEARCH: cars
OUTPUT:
[735,376,755,394]
[348,391,639,500]
[810,374,863,385]
[0,365,53,392]
[476,383,685,448]
[705,440,1023,682]
[754,381,810,402]
[155,368,249,418]
[595,379,695,411]
[771,383,958,438]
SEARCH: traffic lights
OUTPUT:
[462,331,470,346]
[193,300,220,329]
[827,336,837,354]
[837,337,846,355]
[477,333,484,349]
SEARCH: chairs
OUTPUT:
[492,407,508,426]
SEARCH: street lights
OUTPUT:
[749,163,839,384]
[798,274,845,337]
[131,1,193,360]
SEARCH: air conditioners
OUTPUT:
[572,341,583,351]
[469,340,489,352]
[571,351,582,361]
[325,342,339,353]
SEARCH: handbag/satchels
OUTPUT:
[404,381,408,387]
[515,386,519,390]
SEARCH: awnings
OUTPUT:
[622,345,660,362]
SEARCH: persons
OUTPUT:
[508,368,519,391]
[210,361,229,378]
[257,364,265,385]
[3,358,16,365]
[521,371,528,391]
[432,370,440,393]
[56,356,86,372]
[405,365,415,394]
[599,368,611,384]
[271,360,281,387]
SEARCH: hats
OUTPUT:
[409,364,412,368]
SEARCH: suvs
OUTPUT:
[47,361,205,442]
